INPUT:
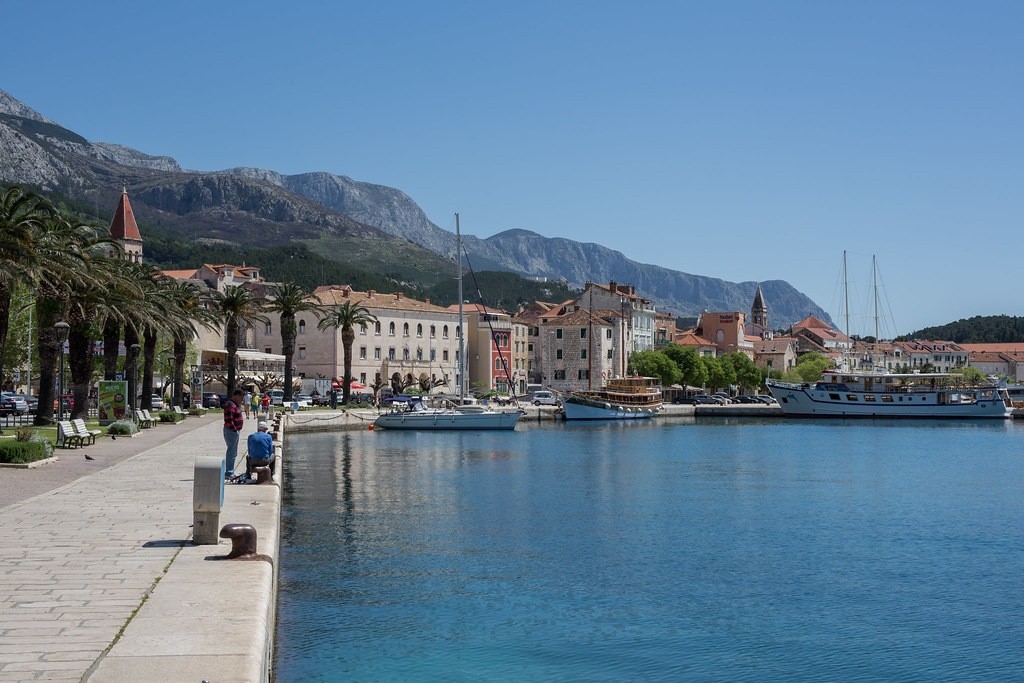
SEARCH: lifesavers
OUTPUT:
[634,370,638,376]
[403,404,408,409]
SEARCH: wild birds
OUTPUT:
[111,434,116,441]
[85,454,95,462]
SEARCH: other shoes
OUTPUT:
[245,473,251,479]
[225,474,236,479]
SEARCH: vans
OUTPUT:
[531,391,562,406]
[268,389,284,404]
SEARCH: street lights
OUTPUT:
[130,343,141,424]
[191,364,197,406]
[168,356,176,411]
[52,322,71,444]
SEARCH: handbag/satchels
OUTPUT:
[257,405,262,411]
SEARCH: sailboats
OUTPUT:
[373,212,527,430]
[546,283,664,420]
[765,249,1014,419]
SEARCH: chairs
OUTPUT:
[173,406,190,419]
[195,404,209,415]
[136,409,161,429]
[55,418,101,449]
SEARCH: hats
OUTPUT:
[258,421,268,430]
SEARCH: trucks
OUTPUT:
[294,379,332,406]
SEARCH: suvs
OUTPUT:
[203,392,226,407]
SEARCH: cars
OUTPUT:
[292,393,313,404]
[672,392,776,405]
[0,392,75,417]
[151,394,164,410]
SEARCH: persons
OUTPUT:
[20,389,24,394]
[223,389,245,480]
[261,393,270,412]
[245,421,275,481]
[252,392,260,419]
[244,391,252,419]
[330,389,337,409]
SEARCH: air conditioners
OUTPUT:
[473,356,480,360]
